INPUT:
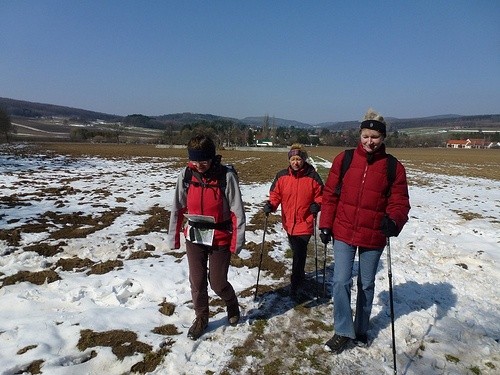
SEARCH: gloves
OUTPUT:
[263,202,272,216]
[381,215,397,237]
[320,228,332,244]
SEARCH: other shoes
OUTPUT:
[289,283,299,296]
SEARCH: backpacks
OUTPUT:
[309,201,320,219]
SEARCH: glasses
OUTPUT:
[190,161,208,166]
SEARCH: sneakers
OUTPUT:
[227,306,240,325]
[187,317,208,340]
[355,332,368,347]
[324,334,350,352]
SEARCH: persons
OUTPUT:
[264,143,324,295]
[319,111,411,354]
[168,136,246,338]
